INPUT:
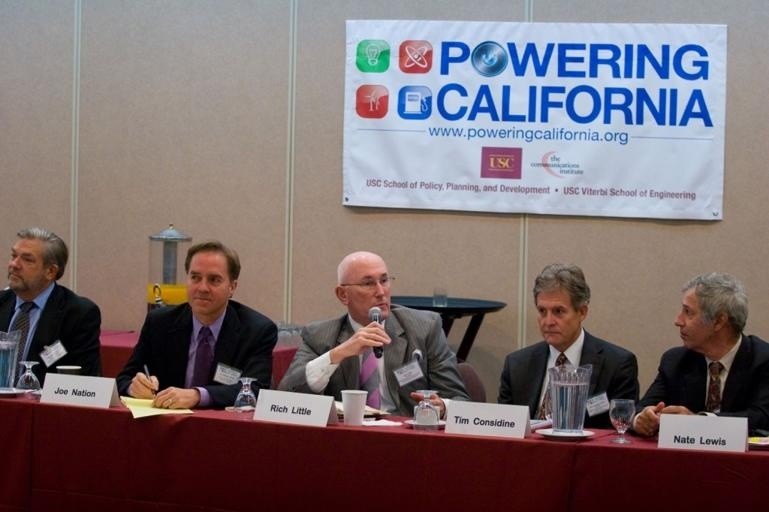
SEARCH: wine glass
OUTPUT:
[17,362,40,401]
[609,399,636,444]
[233,378,258,417]
[414,390,440,433]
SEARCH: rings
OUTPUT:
[168,398,173,404]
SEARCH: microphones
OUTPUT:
[368,307,383,358]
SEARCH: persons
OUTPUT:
[1,226,103,391]
[496,263,642,431]
[619,270,769,438]
[276,251,473,422]
[114,238,278,410]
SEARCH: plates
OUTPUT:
[31,389,43,395]
[0,388,25,396]
[535,429,595,440]
[748,437,769,448]
[404,420,445,429]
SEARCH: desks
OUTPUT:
[0,326,769,512]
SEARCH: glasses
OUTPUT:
[340,273,395,290]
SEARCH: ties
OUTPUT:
[704,362,725,414]
[6,302,35,388]
[189,325,216,391]
[360,349,385,412]
[538,353,568,423]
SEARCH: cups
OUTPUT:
[340,390,368,426]
[433,285,448,307]
[56,366,81,376]
[548,364,593,435]
[0,330,22,393]
